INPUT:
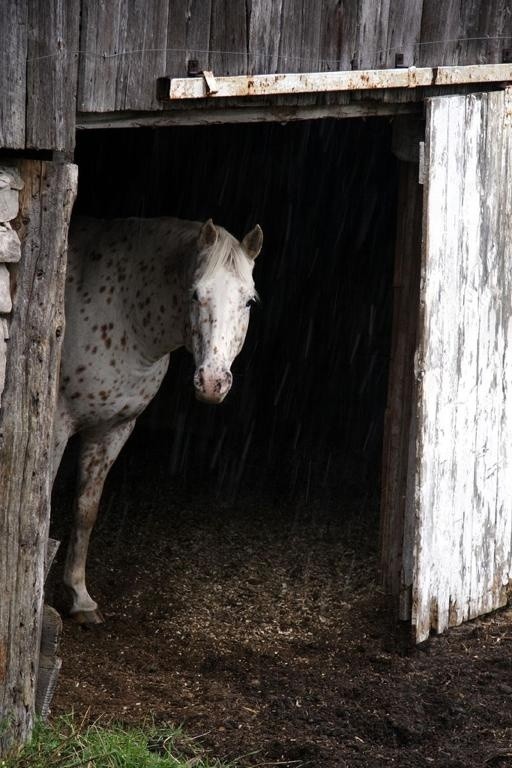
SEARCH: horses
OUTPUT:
[46,216,263,628]
